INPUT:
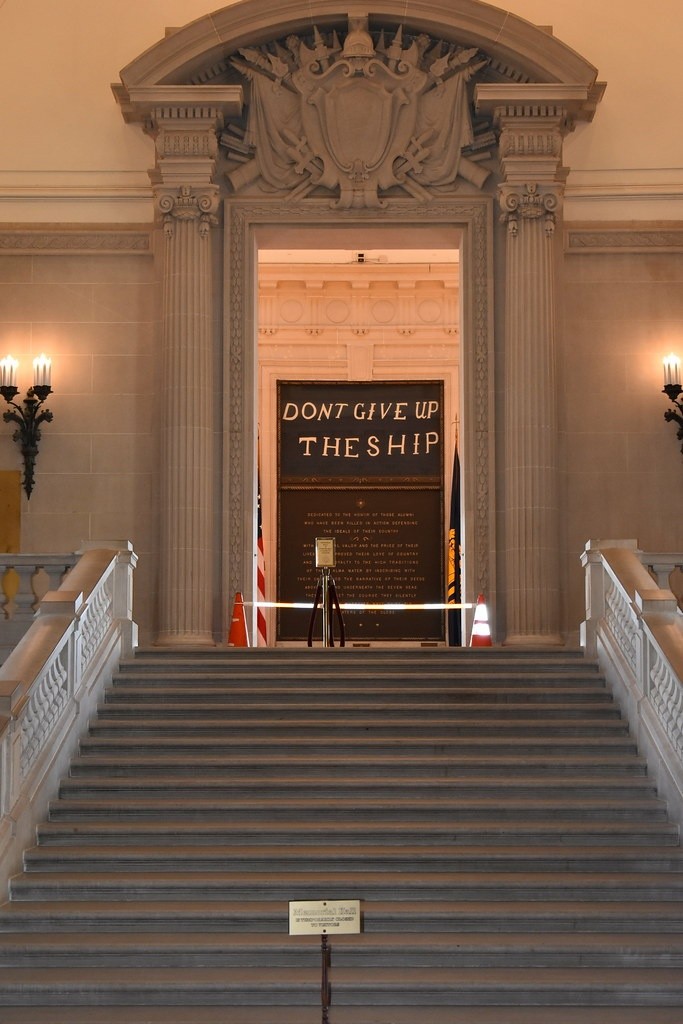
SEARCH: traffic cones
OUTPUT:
[226,593,251,648]
[468,593,494,648]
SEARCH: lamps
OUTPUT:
[661,352,683,457]
[0,351,55,500]
